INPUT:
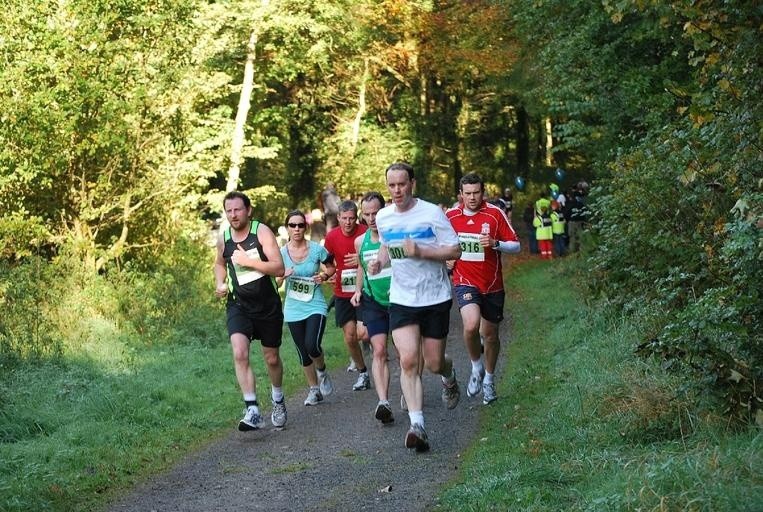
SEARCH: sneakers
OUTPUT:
[303,386,324,406]
[375,401,394,425]
[271,393,287,428]
[351,371,370,391]
[404,423,430,451]
[466,365,486,399]
[318,372,333,398]
[442,375,459,411]
[237,410,265,431]
[348,359,359,373]
[401,394,407,411]
[482,376,498,406]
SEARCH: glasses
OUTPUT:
[287,222,305,229]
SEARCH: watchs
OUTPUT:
[494,240,500,247]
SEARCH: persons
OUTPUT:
[214,192,288,431]
[445,175,521,406]
[350,192,409,424]
[279,202,375,405]
[438,194,462,213]
[484,187,513,224]
[523,178,589,259]
[366,165,462,452]
